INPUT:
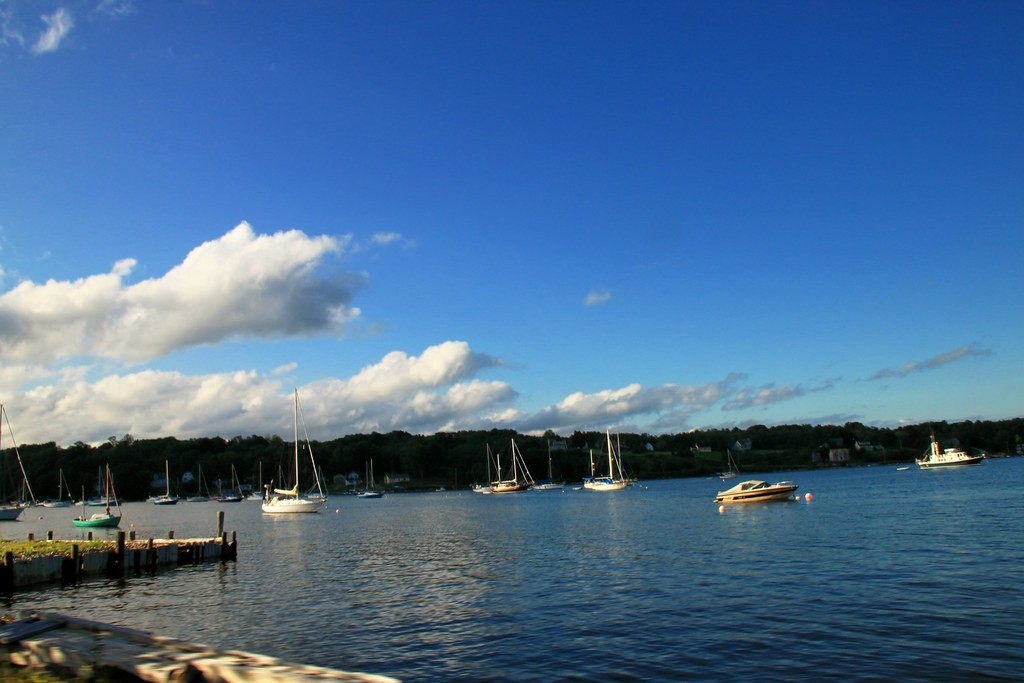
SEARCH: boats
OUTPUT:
[915,434,984,469]
[713,481,799,504]
[154,496,180,505]
[897,466,910,471]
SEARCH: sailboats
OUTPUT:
[187,463,211,502]
[488,439,535,492]
[43,468,70,508]
[533,439,565,490]
[582,429,628,490]
[217,463,245,503]
[146,459,172,504]
[356,459,384,498]
[473,443,501,493]
[261,388,328,514]
[0,403,31,521]
[720,450,739,479]
[72,464,122,528]
[88,465,122,506]
